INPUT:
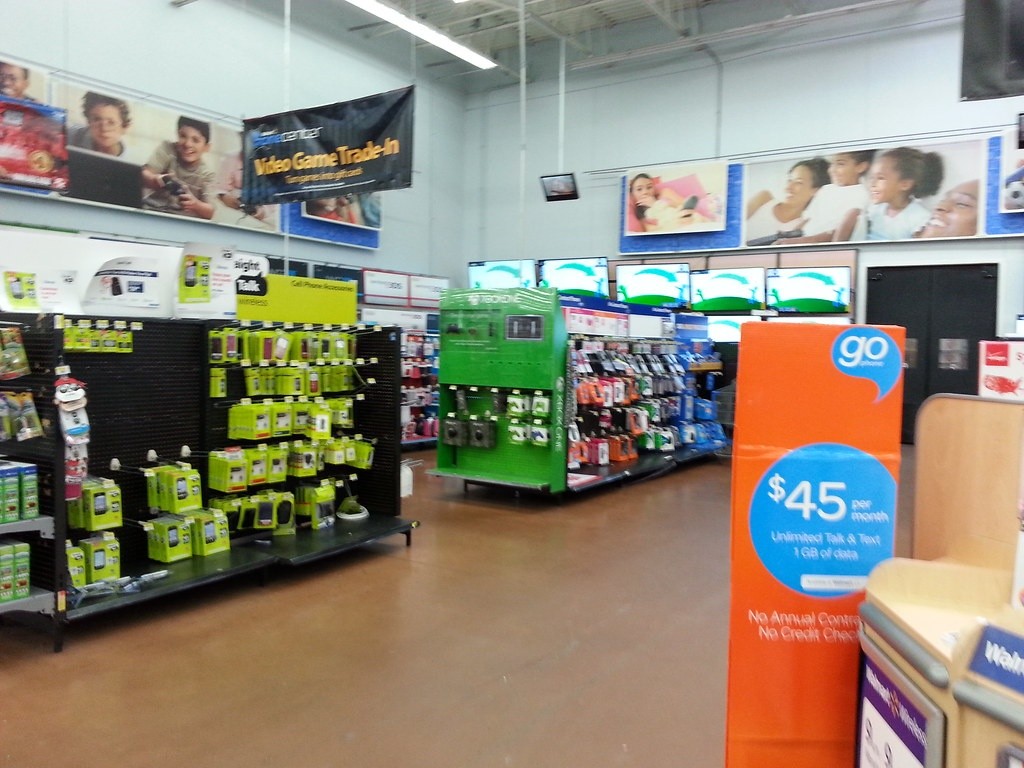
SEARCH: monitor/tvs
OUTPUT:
[541,173,579,202]
[467,257,851,318]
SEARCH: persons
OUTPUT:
[629,171,720,233]
[0,60,279,221]
[744,148,978,245]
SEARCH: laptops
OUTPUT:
[65,145,144,210]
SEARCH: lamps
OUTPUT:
[345,0,499,71]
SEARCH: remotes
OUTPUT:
[747,230,802,246]
[682,197,699,218]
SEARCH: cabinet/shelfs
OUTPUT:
[855,556,1024,768]
[675,337,728,462]
[0,515,55,617]
